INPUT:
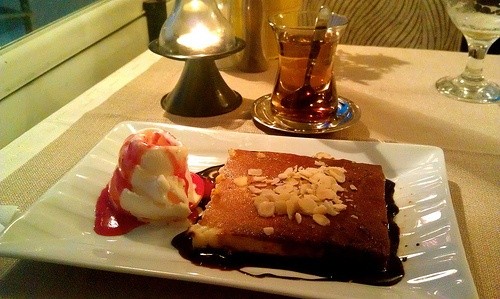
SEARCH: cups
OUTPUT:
[280,6,333,109]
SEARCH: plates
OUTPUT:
[0,121,480,299]
[251,92,361,133]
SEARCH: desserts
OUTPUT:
[187,149,390,257]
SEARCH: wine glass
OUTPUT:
[436,0,500,104]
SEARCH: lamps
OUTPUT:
[144,0,246,117]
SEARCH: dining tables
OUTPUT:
[0,45,500,299]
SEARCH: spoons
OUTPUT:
[269,9,349,123]
